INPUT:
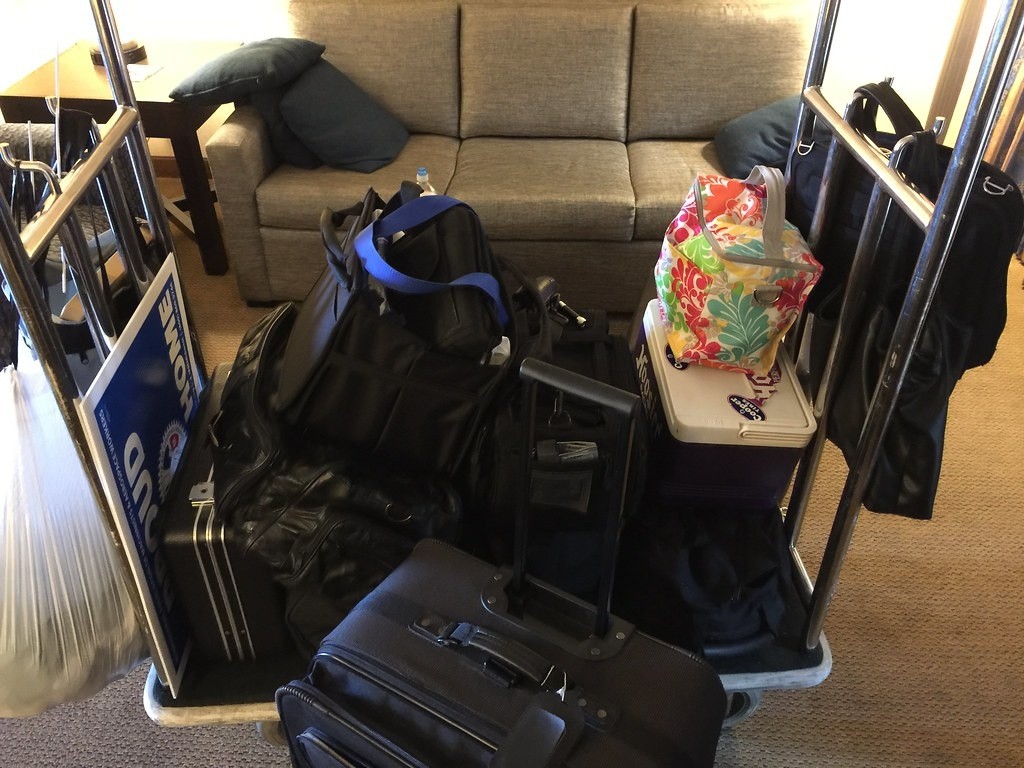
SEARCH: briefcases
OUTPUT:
[154,357,263,672]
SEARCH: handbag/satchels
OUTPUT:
[654,161,823,382]
[809,76,1024,520]
[2,360,157,717]
[460,287,821,673]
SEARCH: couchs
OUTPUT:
[204,0,822,310]
[0,123,110,286]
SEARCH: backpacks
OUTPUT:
[203,178,554,666]
[214,444,443,662]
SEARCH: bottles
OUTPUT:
[416,167,437,197]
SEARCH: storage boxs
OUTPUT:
[631,298,818,513]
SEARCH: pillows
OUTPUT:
[250,90,320,171]
[169,37,326,107]
[277,58,409,173]
[712,93,806,178]
[627,0,822,144]
[287,0,459,139]
[458,0,638,143]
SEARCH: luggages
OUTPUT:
[274,355,726,768]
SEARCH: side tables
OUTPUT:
[0,30,245,278]
